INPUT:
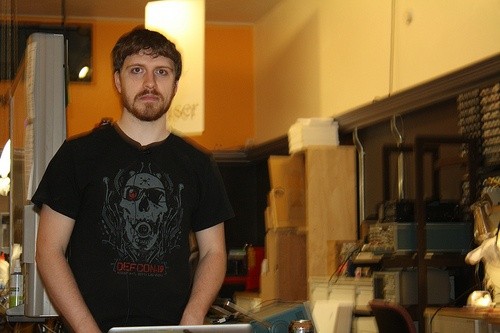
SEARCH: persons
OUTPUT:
[30,28,234,333]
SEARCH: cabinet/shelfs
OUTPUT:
[261,145,357,306]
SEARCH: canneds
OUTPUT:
[288,319,315,333]
[9,272,24,308]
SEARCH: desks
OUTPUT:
[424,308,500,333]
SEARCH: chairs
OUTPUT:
[369,300,417,333]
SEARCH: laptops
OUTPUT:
[108,323,251,333]
